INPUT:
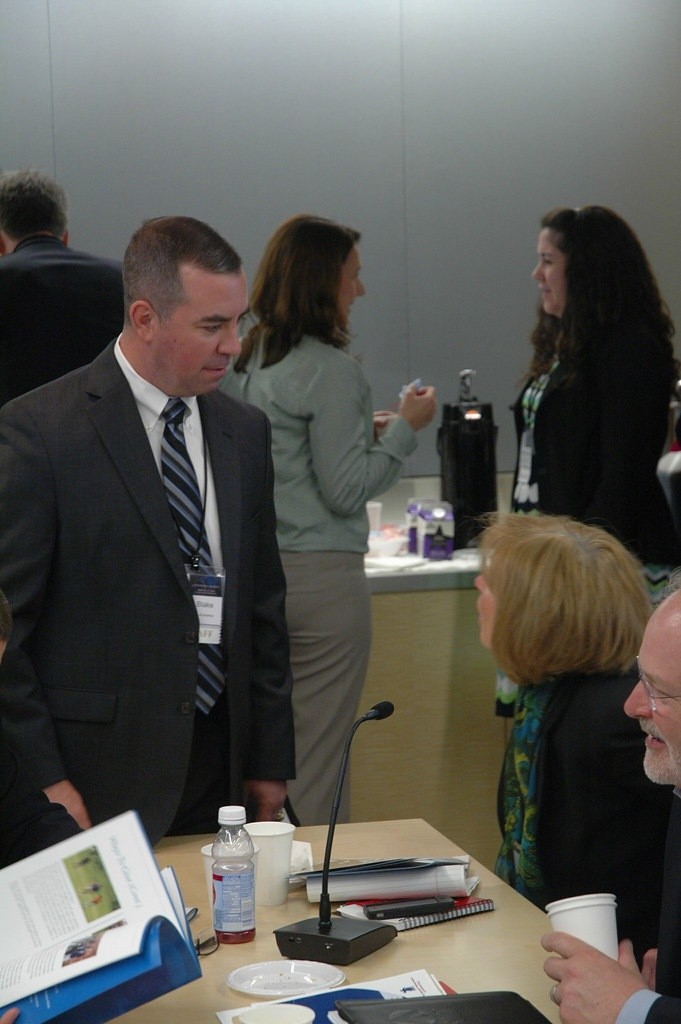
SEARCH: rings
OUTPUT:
[552,984,560,1004]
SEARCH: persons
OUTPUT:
[0,217,296,849]
[0,169,125,406]
[515,203,677,571]
[540,575,681,1024]
[475,513,674,963]
[222,213,437,825]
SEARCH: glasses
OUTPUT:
[636,655,681,711]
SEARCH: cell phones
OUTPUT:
[363,895,455,920]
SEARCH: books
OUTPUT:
[0,809,201,1024]
[338,896,494,931]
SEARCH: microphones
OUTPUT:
[272,700,398,966]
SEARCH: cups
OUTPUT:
[545,894,619,962]
[243,822,296,906]
[201,841,261,907]
[366,500,382,530]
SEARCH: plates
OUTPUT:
[226,959,346,998]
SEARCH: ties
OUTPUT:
[160,398,226,714]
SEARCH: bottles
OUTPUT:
[210,805,257,944]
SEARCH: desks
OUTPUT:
[103,818,564,1024]
[349,547,515,874]
